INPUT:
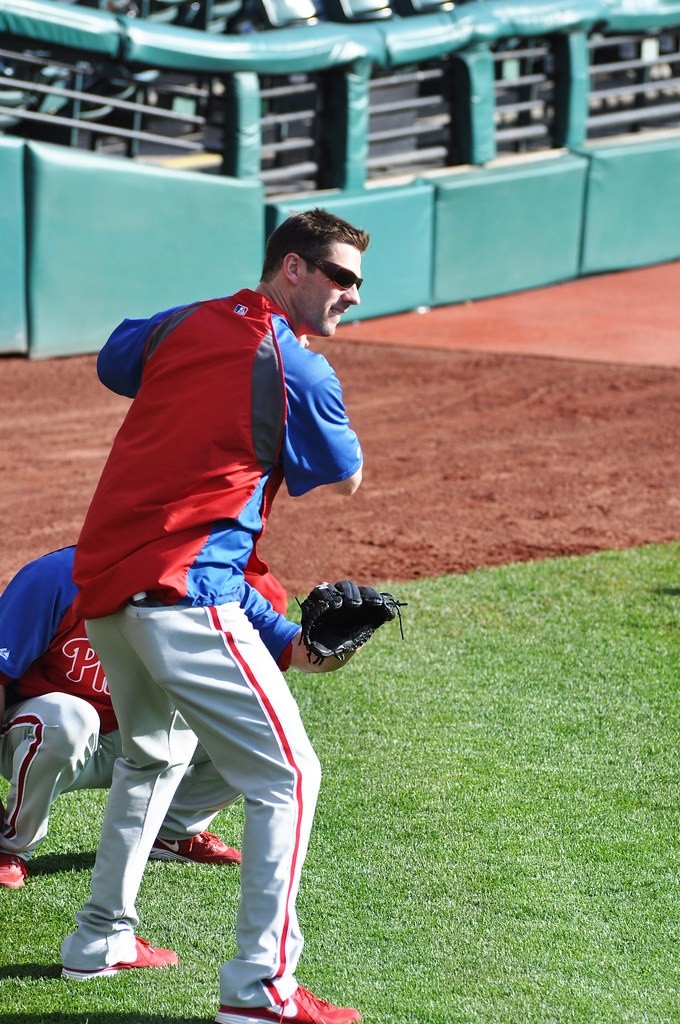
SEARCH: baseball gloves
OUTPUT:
[296,579,408,667]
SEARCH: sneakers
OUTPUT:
[150,833,245,864]
[0,852,30,889]
[217,988,360,1024]
[62,938,181,982]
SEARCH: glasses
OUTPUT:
[294,247,364,290]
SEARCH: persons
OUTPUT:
[61,205,372,1024]
[0,543,407,890]
